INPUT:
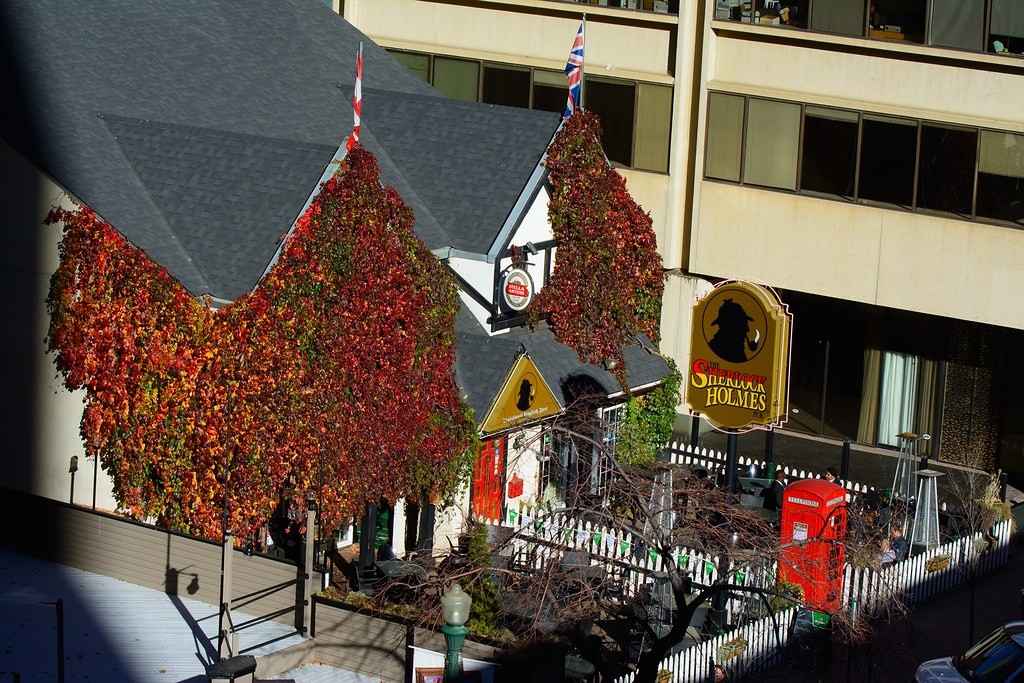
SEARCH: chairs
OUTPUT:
[438,522,772,683]
[348,561,382,597]
[377,544,395,562]
[618,457,786,546]
[416,550,437,574]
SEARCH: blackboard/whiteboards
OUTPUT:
[786,610,831,670]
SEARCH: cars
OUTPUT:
[912,621,1023,683]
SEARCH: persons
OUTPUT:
[843,511,907,571]
[770,471,786,533]
[854,490,880,526]
[715,665,730,683]
[820,466,841,486]
[692,470,721,543]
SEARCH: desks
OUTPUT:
[374,560,425,605]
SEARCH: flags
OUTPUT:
[563,20,583,121]
[346,56,362,151]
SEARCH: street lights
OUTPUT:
[438,582,474,683]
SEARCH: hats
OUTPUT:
[827,466,837,476]
